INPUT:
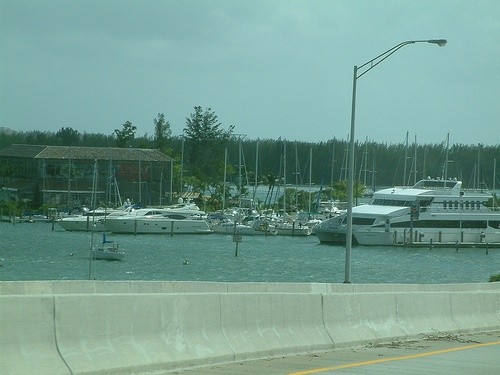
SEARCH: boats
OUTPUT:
[92,234,125,260]
[54,199,134,231]
[99,198,215,234]
[206,200,347,236]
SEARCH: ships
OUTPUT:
[312,176,500,246]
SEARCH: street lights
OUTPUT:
[343,39,450,283]
